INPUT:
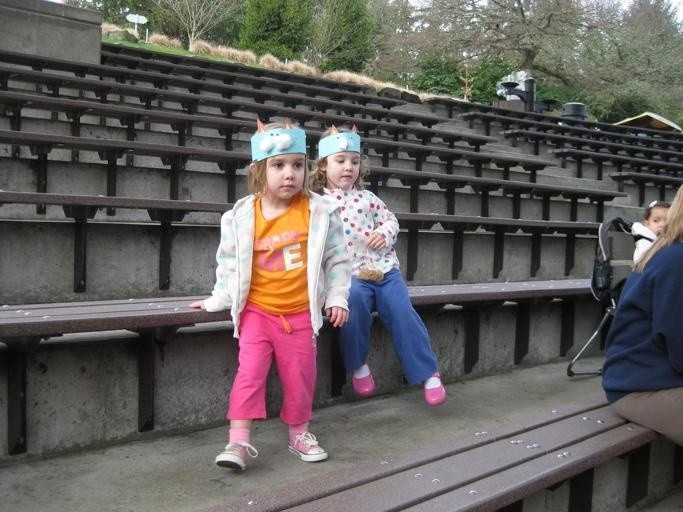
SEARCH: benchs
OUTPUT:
[0,44,682,512]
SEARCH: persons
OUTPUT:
[187,117,352,470]
[631,201,671,262]
[308,124,446,406]
[602,183,683,448]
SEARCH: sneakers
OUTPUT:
[215,440,259,470]
[286,431,328,462]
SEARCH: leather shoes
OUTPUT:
[423,371,447,407]
[351,372,376,398]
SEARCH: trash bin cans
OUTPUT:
[562,101,588,121]
